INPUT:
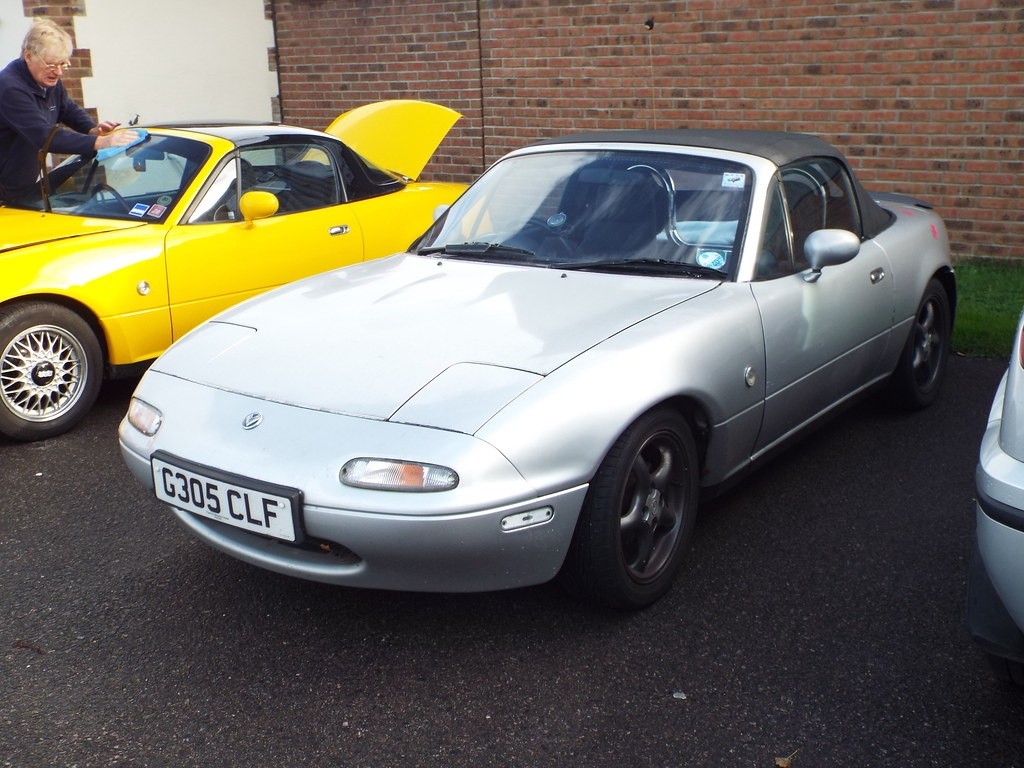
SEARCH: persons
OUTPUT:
[0,20,138,201]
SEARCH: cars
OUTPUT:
[973,312,1024,633]
[0,100,493,440]
[119,128,958,611]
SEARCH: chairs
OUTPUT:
[282,160,332,212]
[566,166,677,256]
[187,155,256,209]
[729,181,822,256]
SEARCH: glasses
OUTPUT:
[35,51,71,72]
